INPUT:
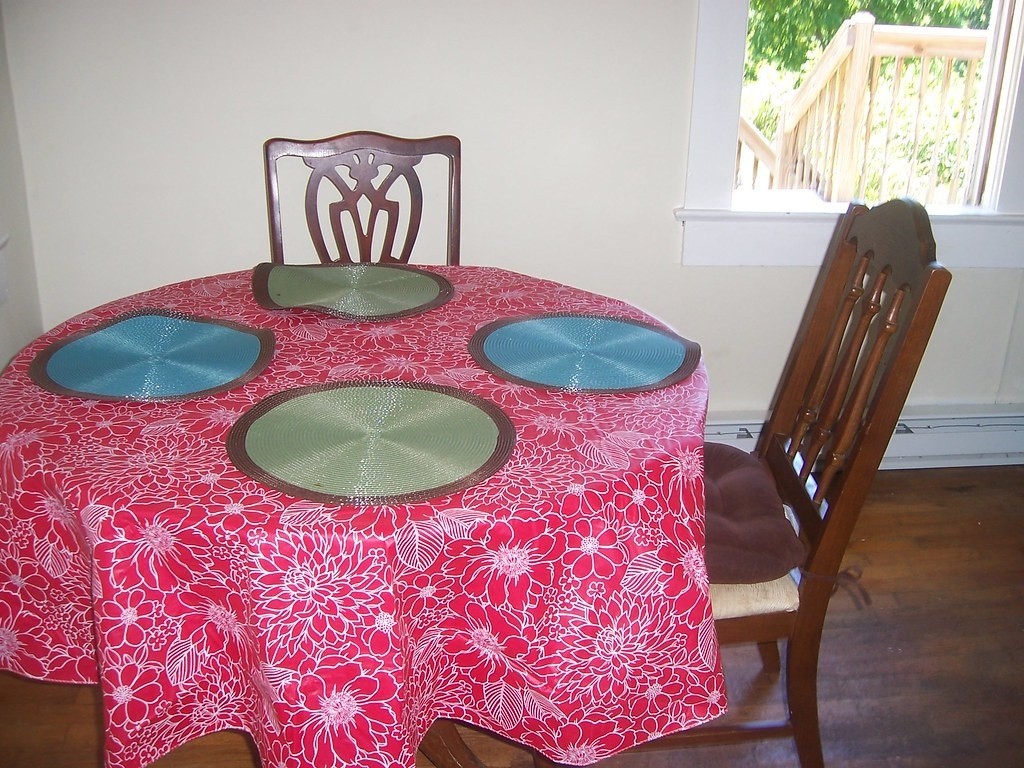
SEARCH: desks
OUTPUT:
[0,262,729,768]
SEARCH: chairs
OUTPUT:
[264,130,462,265]
[534,199,951,768]
